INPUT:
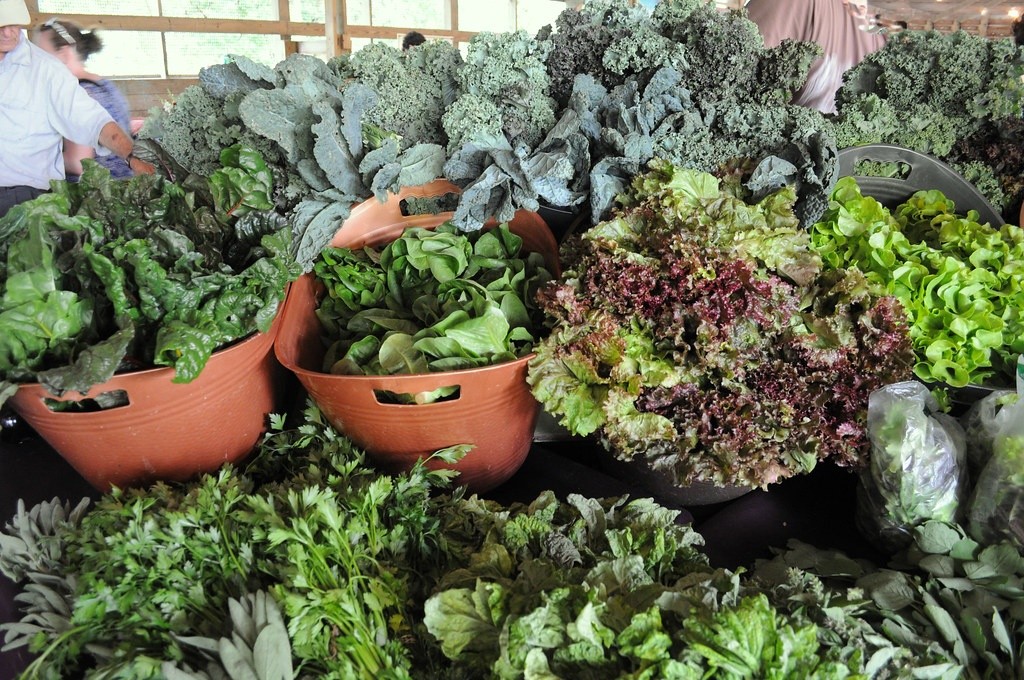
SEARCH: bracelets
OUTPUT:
[125,153,140,170]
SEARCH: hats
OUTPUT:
[0,0,31,28]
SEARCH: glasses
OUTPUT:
[44,16,76,44]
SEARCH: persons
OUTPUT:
[32,20,134,182]
[0,0,158,218]
[403,31,426,52]
[876,14,907,43]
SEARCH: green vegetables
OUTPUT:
[0,0,1024,680]
